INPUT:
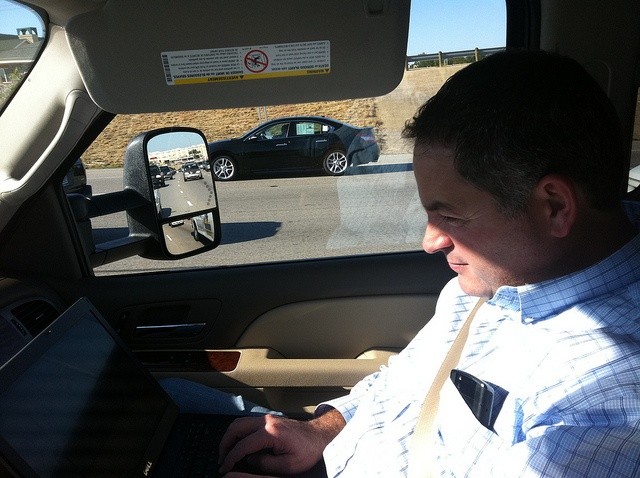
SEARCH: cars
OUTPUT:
[168,219,184,228]
[190,211,215,242]
[207,115,380,180]
[149,157,209,188]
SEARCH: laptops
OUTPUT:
[0,293,274,478]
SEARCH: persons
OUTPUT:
[157,50,640,478]
